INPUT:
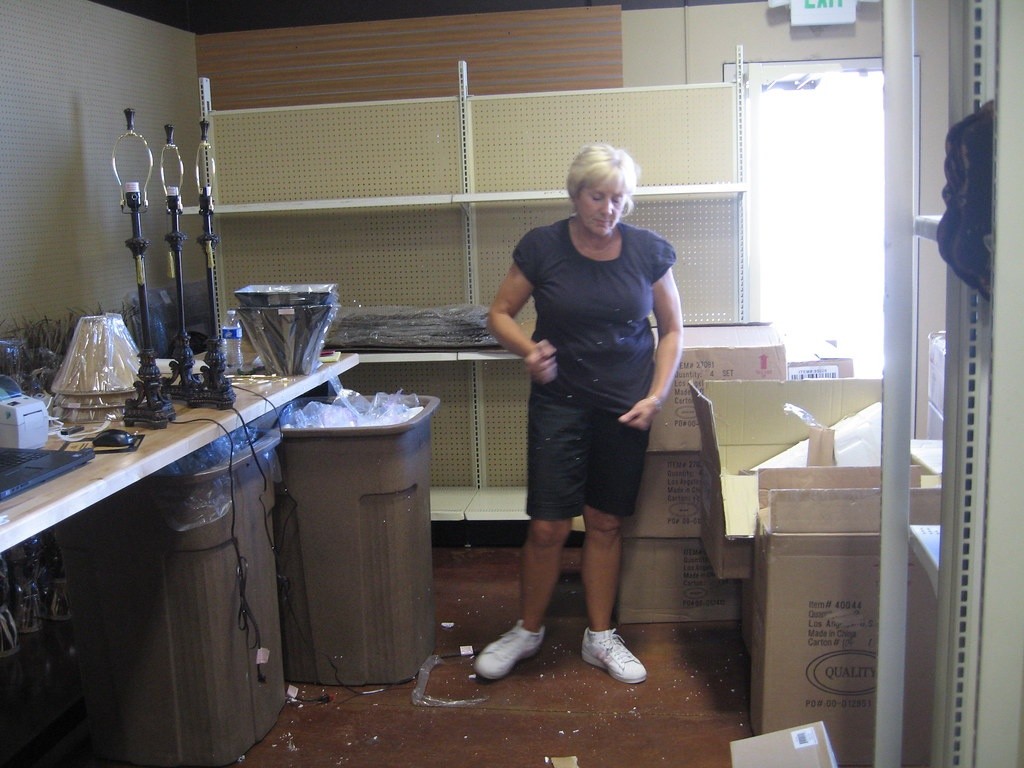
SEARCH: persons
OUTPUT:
[472,137,685,685]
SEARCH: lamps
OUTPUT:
[233,284,341,376]
[186,118,237,411]
[161,124,199,402]
[112,107,177,429]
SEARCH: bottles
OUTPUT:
[221,310,243,374]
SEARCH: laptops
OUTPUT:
[0,448,96,503]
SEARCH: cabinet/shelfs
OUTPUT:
[198,45,746,521]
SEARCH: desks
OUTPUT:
[0,352,359,556]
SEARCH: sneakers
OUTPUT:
[581,627,647,683]
[474,619,545,679]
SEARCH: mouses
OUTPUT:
[92,429,135,447]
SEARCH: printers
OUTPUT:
[0,374,48,448]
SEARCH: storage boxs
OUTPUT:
[927,401,944,439]
[620,451,705,539]
[687,378,881,580]
[614,539,740,623]
[645,320,786,453]
[729,721,838,768]
[749,466,942,768]
[927,330,947,416]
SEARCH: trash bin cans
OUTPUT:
[274,392,443,684]
[58,431,285,768]
